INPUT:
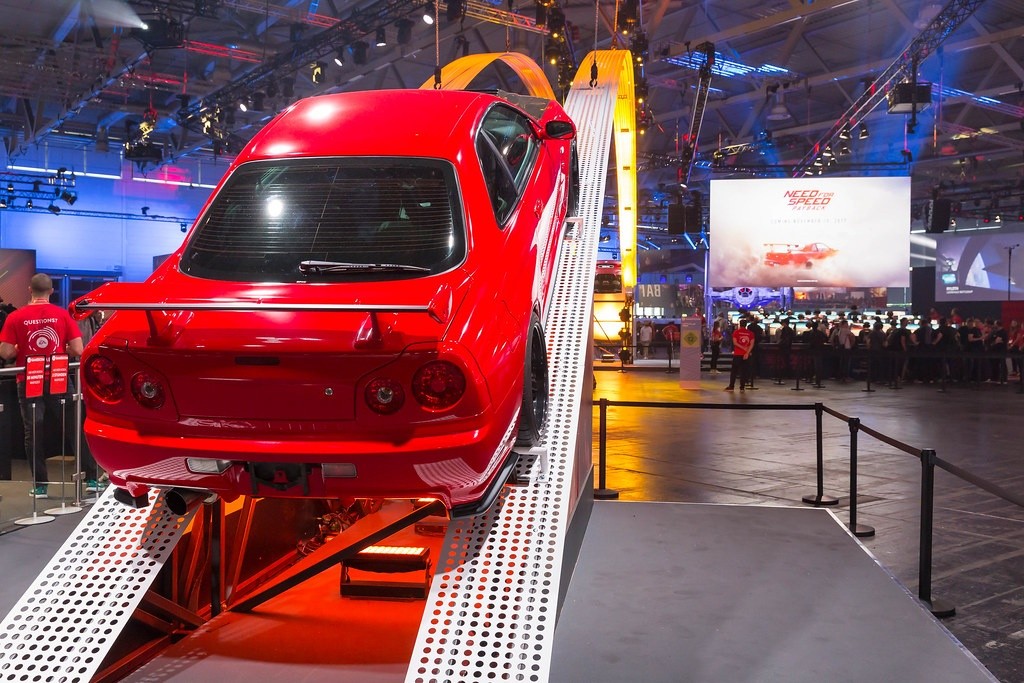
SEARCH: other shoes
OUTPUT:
[740,387,744,391]
[897,375,1008,385]
[726,387,734,391]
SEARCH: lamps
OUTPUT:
[422,2,435,24]
[376,25,387,48]
[804,120,869,175]
[679,181,688,190]
[240,95,250,112]
[334,50,345,66]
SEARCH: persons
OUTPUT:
[725,307,1024,392]
[0,273,105,498]
[636,307,730,375]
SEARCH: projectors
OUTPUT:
[124,146,162,162]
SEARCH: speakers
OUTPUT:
[922,198,951,234]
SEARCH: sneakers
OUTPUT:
[85,479,106,492]
[29,484,48,499]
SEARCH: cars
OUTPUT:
[764,241,840,270]
[67,87,581,523]
